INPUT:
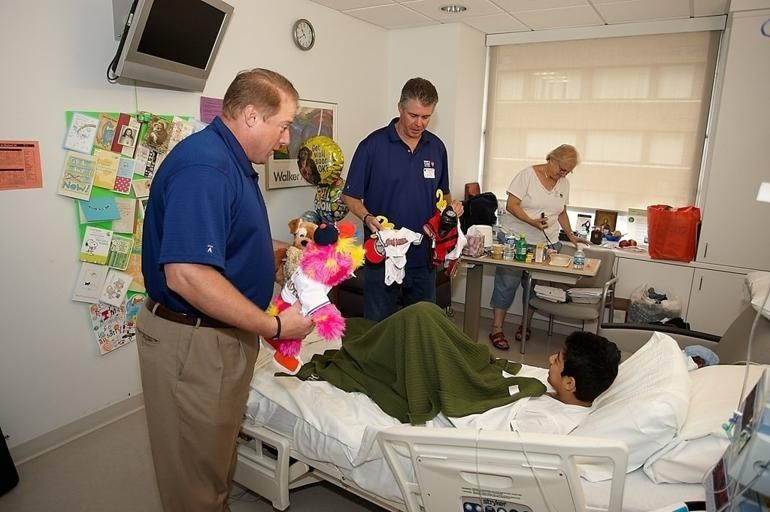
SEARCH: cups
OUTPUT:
[464,233,486,257]
[492,244,503,260]
[591,230,602,245]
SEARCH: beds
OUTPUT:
[230,272,770,511]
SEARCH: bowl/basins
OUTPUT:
[605,233,625,241]
[684,344,720,366]
[550,253,571,265]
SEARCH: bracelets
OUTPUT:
[363,213,372,223]
[269,314,280,341]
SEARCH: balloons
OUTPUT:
[315,178,351,223]
[297,136,345,188]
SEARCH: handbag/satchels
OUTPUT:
[647,205,700,262]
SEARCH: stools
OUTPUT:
[605,296,631,323]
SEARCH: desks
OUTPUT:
[458,254,602,344]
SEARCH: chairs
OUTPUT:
[520,241,617,357]
[327,266,453,319]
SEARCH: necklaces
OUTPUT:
[544,169,557,192]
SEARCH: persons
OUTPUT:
[119,128,134,147]
[601,218,611,232]
[146,121,167,147]
[579,221,590,234]
[342,77,465,324]
[490,144,595,350]
[135,68,315,512]
[422,330,620,436]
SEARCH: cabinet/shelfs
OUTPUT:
[583,247,750,337]
[696,10,770,270]
[383,24,486,203]
[450,259,583,337]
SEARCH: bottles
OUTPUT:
[577,226,587,240]
[505,224,526,261]
[600,217,610,235]
[438,210,457,237]
[573,245,586,269]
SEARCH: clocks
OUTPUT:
[292,19,316,51]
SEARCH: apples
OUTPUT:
[628,239,637,246]
[619,240,629,247]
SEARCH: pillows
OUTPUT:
[565,331,692,481]
[643,360,764,483]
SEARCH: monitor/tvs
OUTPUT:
[112,0,234,93]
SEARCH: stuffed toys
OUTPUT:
[262,222,362,377]
[276,218,320,290]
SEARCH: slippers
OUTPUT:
[489,333,509,350]
[515,326,531,341]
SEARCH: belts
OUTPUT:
[145,296,236,328]
